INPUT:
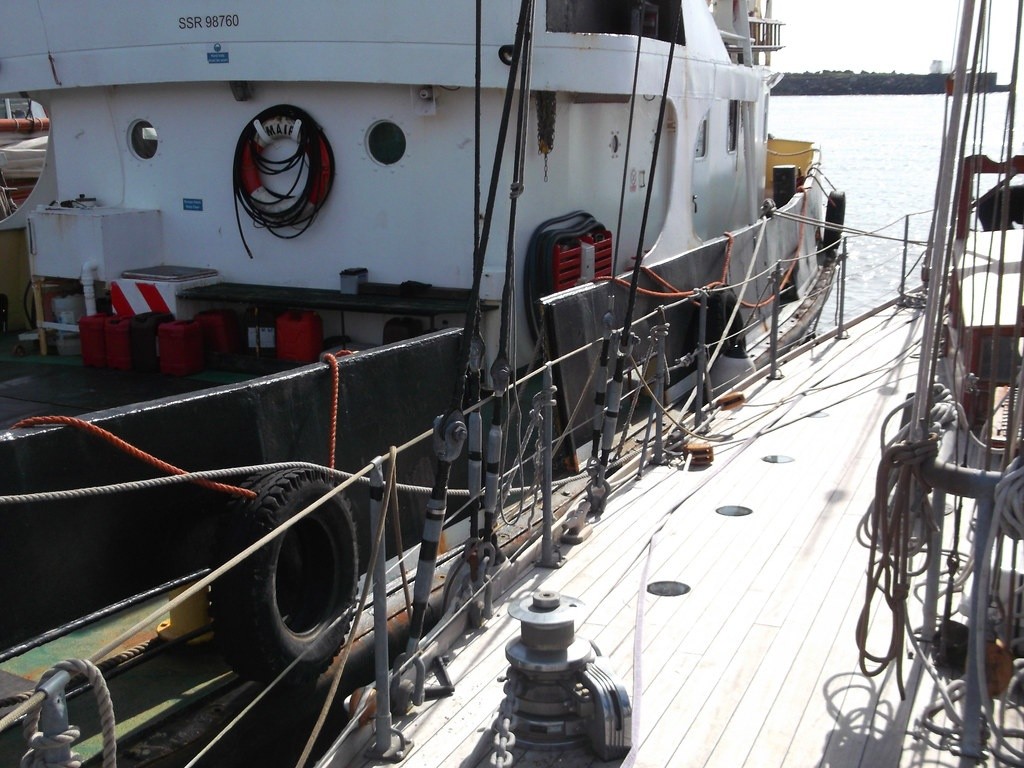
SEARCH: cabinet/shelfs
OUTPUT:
[26,207,162,355]
[110,275,220,323]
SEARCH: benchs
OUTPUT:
[175,282,499,360]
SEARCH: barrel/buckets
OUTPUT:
[158,320,204,374]
[106,316,131,369]
[383,317,422,345]
[193,308,239,352]
[80,312,105,369]
[276,308,324,363]
[242,307,275,357]
[131,312,173,372]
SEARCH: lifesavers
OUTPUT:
[821,188,846,253]
[205,458,364,694]
[230,102,336,229]
[707,280,746,361]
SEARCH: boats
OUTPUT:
[0,0,1024,767]
[0,1,837,768]
